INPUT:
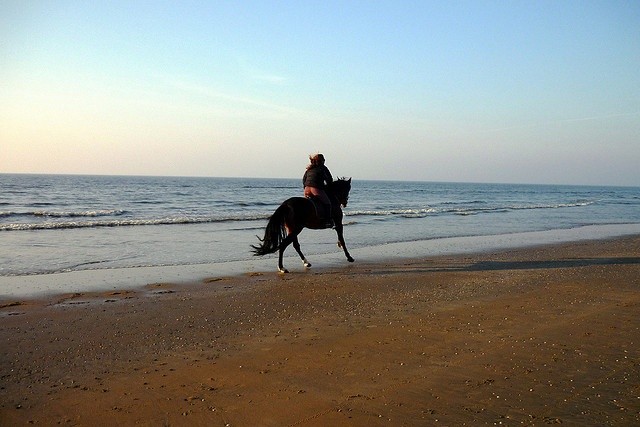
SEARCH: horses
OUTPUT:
[248,176,355,273]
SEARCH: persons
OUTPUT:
[302,154,333,220]
